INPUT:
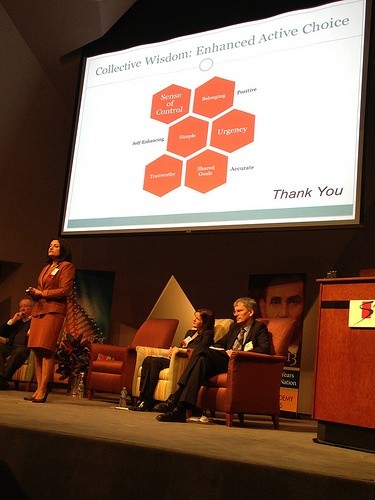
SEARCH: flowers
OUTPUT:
[56,336,91,377]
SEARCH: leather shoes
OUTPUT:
[155,394,177,412]
[156,408,186,421]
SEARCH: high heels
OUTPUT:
[132,400,154,411]
[32,389,50,402]
[129,400,140,410]
[24,396,36,400]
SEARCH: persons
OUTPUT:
[258,273,305,369]
[128,308,216,413]
[153,297,270,424]
[0,295,34,386]
[23,238,77,404]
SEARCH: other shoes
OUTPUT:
[0,381,9,389]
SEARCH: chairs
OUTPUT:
[197,316,301,429]
[85,319,178,409]
[9,348,36,391]
[133,319,232,411]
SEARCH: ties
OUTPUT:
[234,328,246,351]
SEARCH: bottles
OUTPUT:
[119,387,127,408]
[77,381,84,400]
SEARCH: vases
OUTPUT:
[69,372,86,398]
